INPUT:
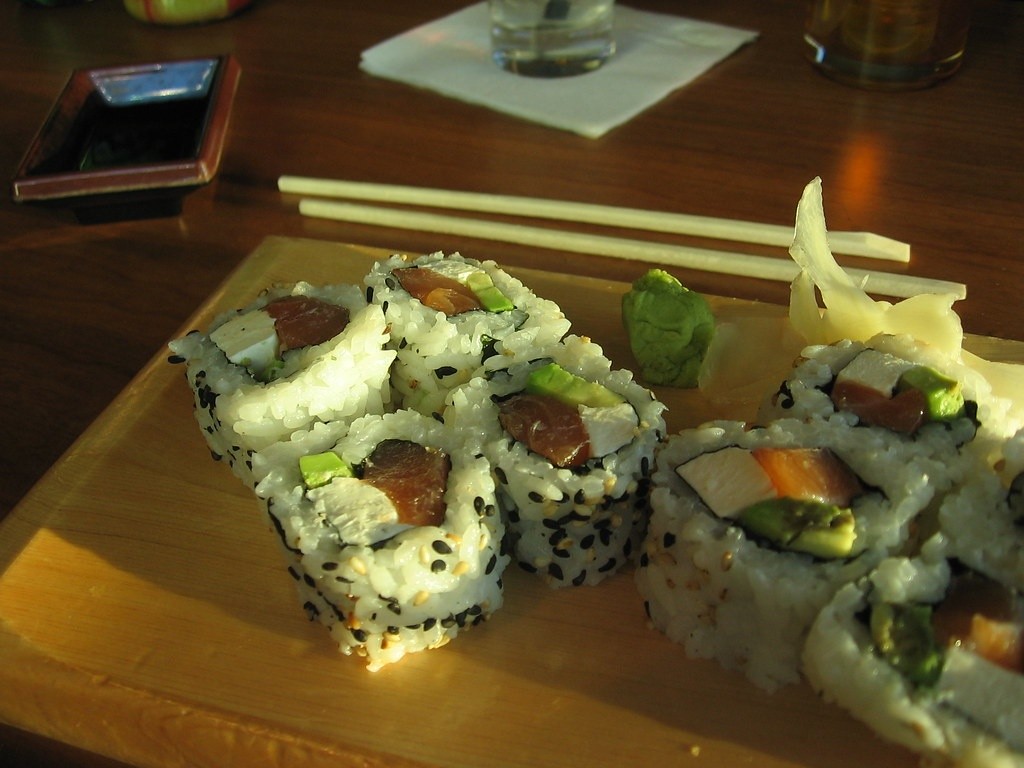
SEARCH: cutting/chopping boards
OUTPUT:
[0,230,1024,768]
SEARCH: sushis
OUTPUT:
[164,250,1024,768]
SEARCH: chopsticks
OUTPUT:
[273,175,967,303]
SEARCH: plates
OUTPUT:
[11,51,243,203]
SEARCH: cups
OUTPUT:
[803,0,973,92]
[486,0,616,80]
[120,0,251,29]
